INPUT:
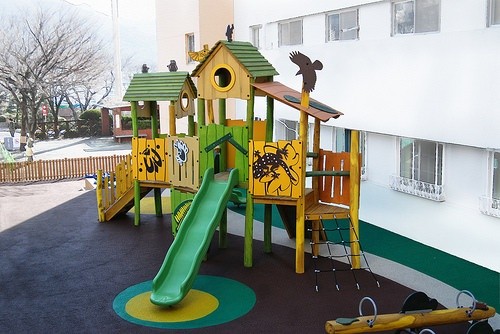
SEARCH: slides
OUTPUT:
[150,167,240,307]
